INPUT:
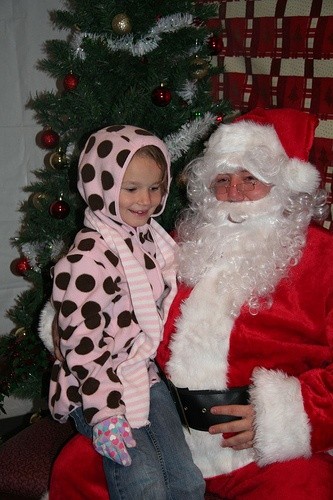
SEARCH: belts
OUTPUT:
[164,377,257,435]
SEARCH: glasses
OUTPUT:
[209,178,259,194]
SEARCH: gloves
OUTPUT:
[92,414,137,467]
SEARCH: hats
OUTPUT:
[199,107,322,196]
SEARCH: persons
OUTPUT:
[47,123,210,500]
[48,100,333,500]
